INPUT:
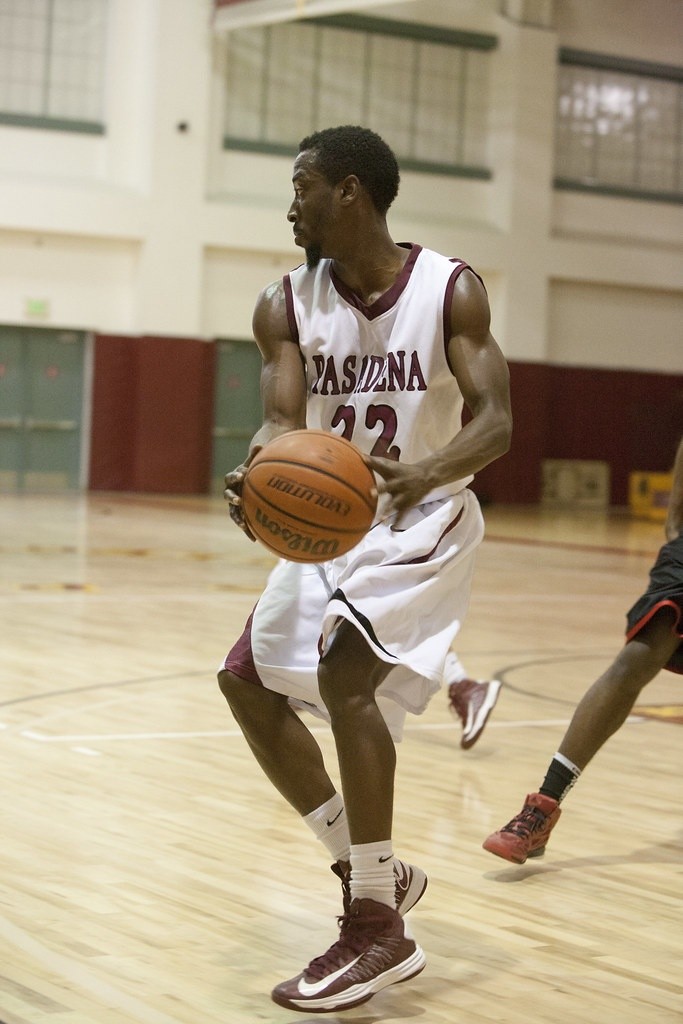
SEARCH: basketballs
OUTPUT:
[239,428,380,564]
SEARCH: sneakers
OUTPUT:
[271,899,426,1013]
[449,679,499,749]
[330,849,427,916]
[482,793,562,864]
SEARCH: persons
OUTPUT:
[217,125,513,1015]
[482,432,683,863]
[443,645,502,752]
